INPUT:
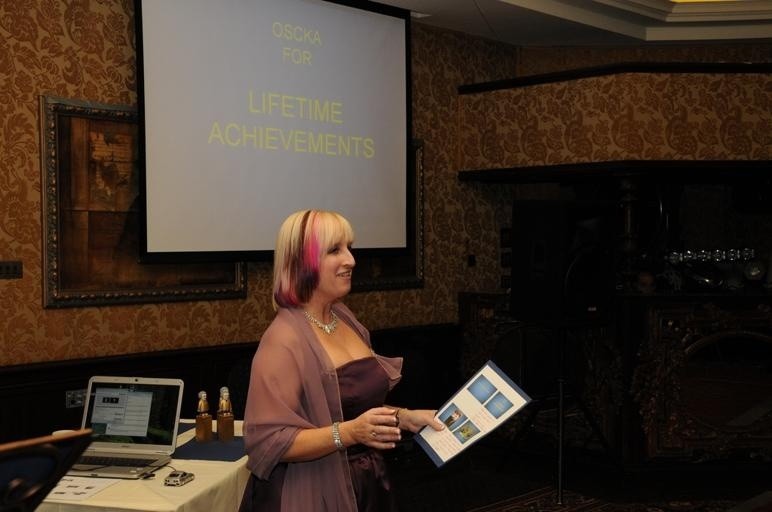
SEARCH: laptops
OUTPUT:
[65,375,185,479]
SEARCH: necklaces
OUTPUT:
[301,307,338,335]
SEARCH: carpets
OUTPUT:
[469,482,772,512]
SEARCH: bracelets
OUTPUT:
[395,407,405,425]
[331,421,346,451]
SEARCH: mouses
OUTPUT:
[164,471,194,487]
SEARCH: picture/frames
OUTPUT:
[39,94,251,309]
[350,137,426,294]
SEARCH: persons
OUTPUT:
[242,209,446,512]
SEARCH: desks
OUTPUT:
[42,418,263,512]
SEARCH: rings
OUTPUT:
[370,432,377,440]
